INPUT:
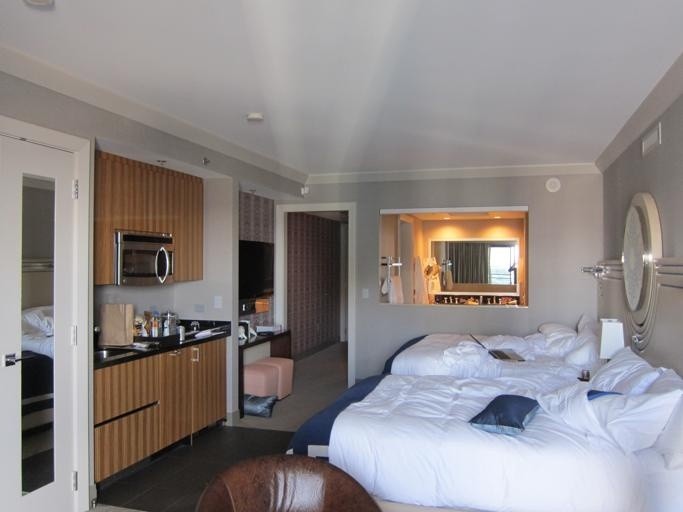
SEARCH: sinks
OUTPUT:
[94,349,137,365]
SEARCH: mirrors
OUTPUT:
[20,170,55,495]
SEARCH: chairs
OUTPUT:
[198,453,382,511]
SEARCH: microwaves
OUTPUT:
[114,230,175,285]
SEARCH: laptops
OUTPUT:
[469,334,526,362]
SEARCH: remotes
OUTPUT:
[258,332,273,336]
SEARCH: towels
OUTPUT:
[381,276,404,304]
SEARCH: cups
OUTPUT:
[582,370,590,380]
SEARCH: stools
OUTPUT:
[245,364,279,398]
[259,356,293,399]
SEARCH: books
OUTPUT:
[489,348,525,362]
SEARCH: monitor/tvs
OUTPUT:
[239,240,275,299]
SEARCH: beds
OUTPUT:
[384,330,615,374]
[288,371,683,512]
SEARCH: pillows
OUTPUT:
[640,370,683,466]
[468,394,545,436]
[592,347,656,397]
[540,320,597,366]
[244,394,274,417]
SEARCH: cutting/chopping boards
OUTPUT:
[97,303,134,347]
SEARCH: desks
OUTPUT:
[238,325,292,417]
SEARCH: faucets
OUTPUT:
[449,297,453,303]
[94,325,101,335]
[493,296,496,305]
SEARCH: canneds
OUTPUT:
[175,325,185,343]
[151,320,158,338]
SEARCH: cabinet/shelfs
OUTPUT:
[93,336,228,486]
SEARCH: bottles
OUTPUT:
[142,310,185,343]
